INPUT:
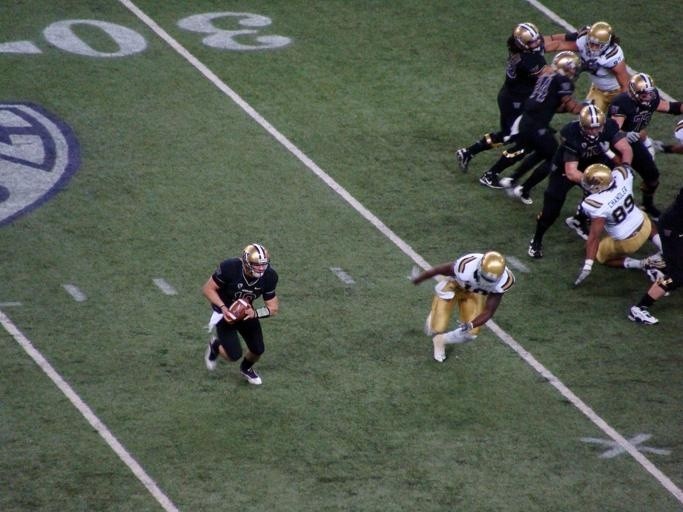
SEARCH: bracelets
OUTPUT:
[255,306,271,319]
[219,304,225,309]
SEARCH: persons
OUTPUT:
[457,19,682,325]
[406,250,516,364]
[202,243,278,385]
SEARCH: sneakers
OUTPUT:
[628,252,669,325]
[457,148,533,204]
[433,334,446,362]
[529,240,543,259]
[241,367,262,385]
[205,337,219,370]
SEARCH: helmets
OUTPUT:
[579,105,606,143]
[581,164,614,194]
[553,22,613,78]
[243,244,270,278]
[514,22,541,50]
[481,252,506,282]
[627,73,655,107]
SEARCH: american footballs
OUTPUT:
[227,299,249,321]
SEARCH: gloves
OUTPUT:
[627,131,640,144]
[574,269,591,285]
